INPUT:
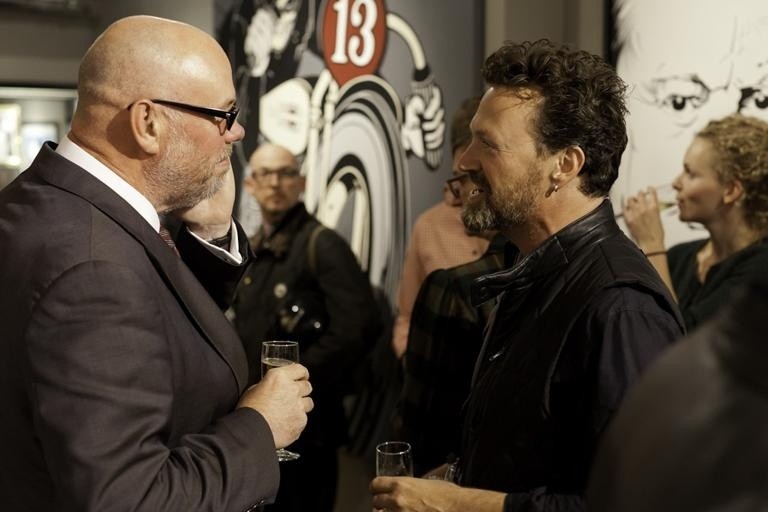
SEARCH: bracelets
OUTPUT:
[206,234,233,248]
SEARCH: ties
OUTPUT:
[158,223,182,259]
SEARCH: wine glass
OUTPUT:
[257,338,305,464]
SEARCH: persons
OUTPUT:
[608,2,767,249]
[582,252,766,509]
[3,13,321,511]
[363,35,685,510]
[393,201,488,364]
[398,93,522,475]
[238,142,391,511]
[621,113,767,305]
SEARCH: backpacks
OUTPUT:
[307,225,395,462]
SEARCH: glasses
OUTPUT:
[126,99,240,131]
[252,166,300,183]
[447,174,469,199]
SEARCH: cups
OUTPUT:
[374,439,413,484]
[656,184,679,213]
